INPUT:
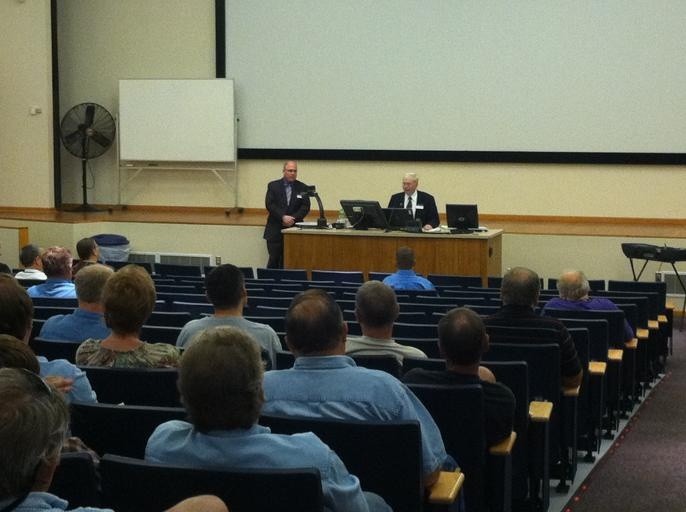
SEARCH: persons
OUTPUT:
[542,272,635,342]
[144,325,391,511]
[382,246,435,293]
[388,175,440,230]
[262,286,465,511]
[175,264,282,368]
[346,279,427,362]
[0,368,227,512]
[1,238,173,454]
[263,160,311,268]
[479,266,583,386]
[403,308,516,433]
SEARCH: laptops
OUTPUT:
[446,204,487,234]
[382,208,421,233]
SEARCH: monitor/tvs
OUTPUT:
[340,199,389,230]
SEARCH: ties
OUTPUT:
[286,185,292,206]
[406,196,413,218]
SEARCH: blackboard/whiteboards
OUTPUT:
[118,78,237,163]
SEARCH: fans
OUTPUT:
[59,101,116,213]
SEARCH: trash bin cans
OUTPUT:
[93,234,129,262]
